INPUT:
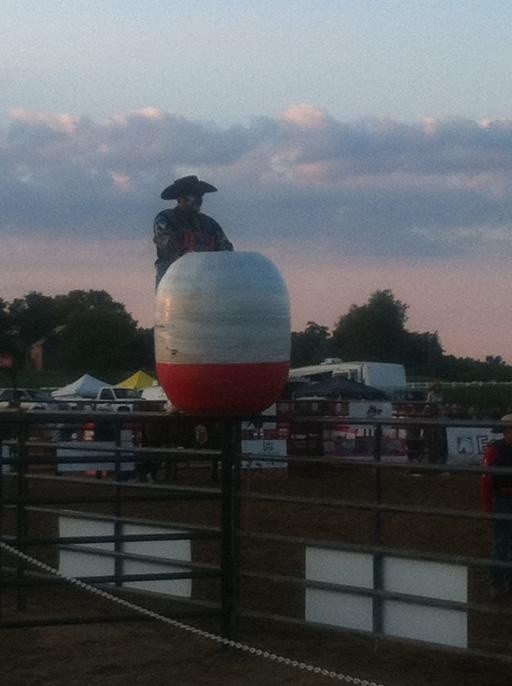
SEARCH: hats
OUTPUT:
[491,413,512,433]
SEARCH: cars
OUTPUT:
[0,387,72,413]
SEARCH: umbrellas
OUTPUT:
[290,374,382,401]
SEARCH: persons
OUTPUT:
[422,376,450,463]
[151,173,234,295]
[481,412,511,598]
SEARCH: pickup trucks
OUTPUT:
[54,386,163,413]
